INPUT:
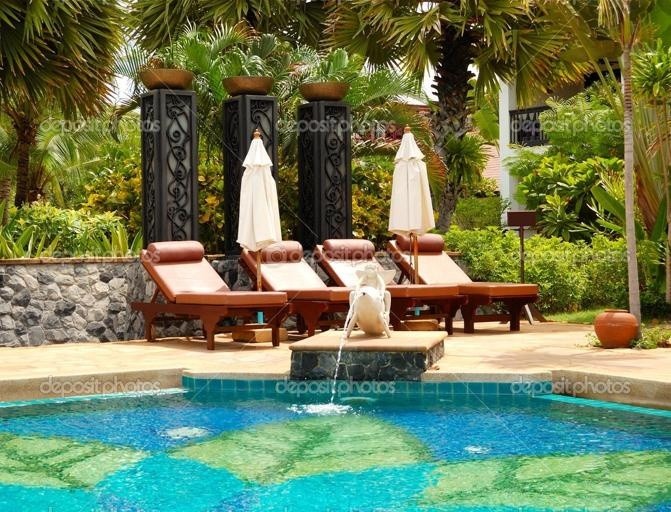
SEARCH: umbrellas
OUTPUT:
[388,126,436,284]
[236,128,283,293]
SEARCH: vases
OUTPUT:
[595,309,637,347]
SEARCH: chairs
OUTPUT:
[132,234,537,350]
